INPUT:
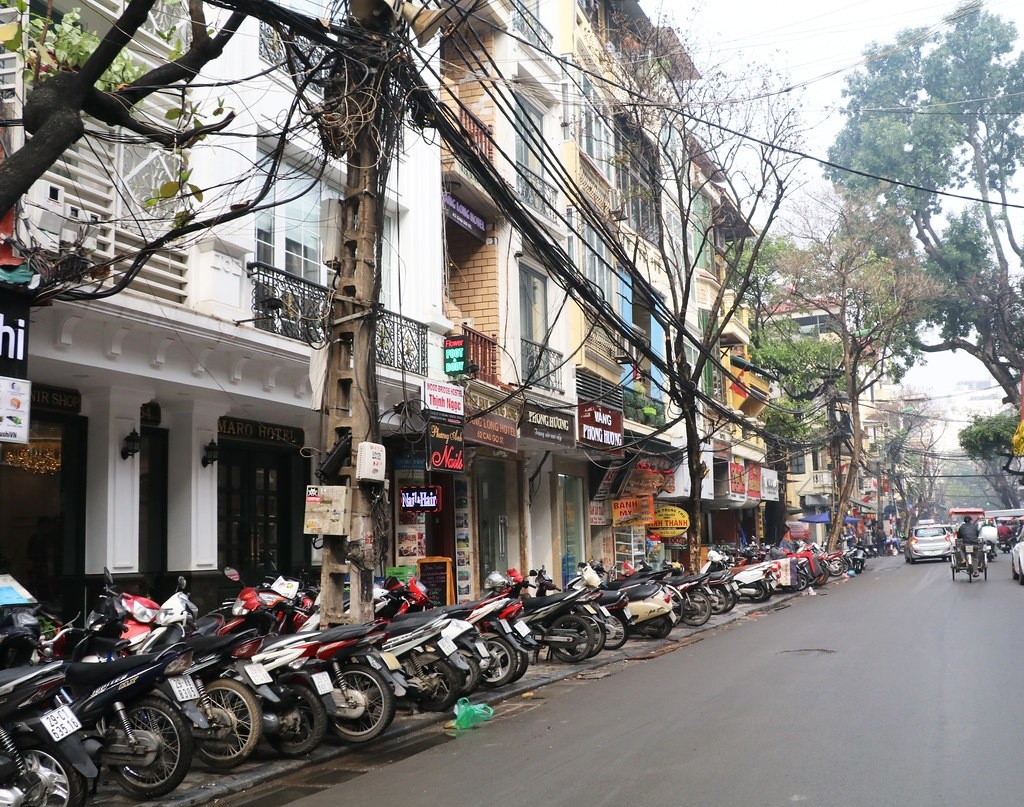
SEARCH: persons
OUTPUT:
[958,515,980,577]
[877,524,886,557]
[737,522,747,547]
[997,521,1013,549]
[1011,521,1024,542]
[822,523,858,548]
[872,521,877,543]
[982,519,998,557]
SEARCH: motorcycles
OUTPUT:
[0,539,852,807]
[852,544,868,574]
[986,540,996,562]
[999,535,1009,553]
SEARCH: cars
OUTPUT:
[902,519,957,564]
[1008,525,1024,585]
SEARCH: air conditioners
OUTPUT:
[615,331,636,363]
[609,187,628,221]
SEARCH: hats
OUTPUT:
[964,516,973,522]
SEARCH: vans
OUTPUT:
[984,509,1024,527]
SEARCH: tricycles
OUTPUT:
[951,537,988,583]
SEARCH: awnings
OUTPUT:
[848,496,879,510]
[861,512,877,514]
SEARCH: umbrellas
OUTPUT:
[798,507,861,523]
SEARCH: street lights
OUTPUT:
[874,408,914,526]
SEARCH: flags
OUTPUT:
[883,479,889,492]
[861,496,871,502]
[833,465,845,476]
[729,371,747,398]
